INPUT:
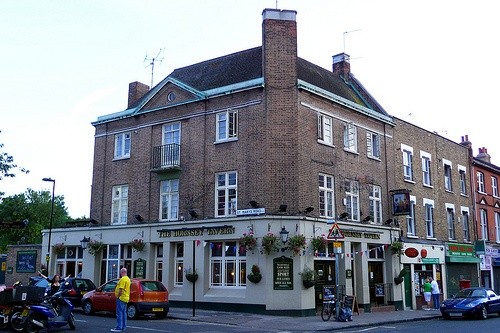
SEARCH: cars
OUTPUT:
[439,287,500,320]
[80,278,170,320]
[32,276,98,304]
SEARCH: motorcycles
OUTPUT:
[23,288,76,333]
[9,290,58,332]
[0,281,27,330]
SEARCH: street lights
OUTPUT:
[42,177,55,277]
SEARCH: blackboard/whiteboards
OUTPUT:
[344,295,359,313]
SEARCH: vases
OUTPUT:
[249,277,261,283]
[133,245,144,252]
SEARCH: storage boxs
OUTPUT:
[0,285,46,305]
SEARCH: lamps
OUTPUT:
[80,235,90,250]
[249,200,260,207]
[341,212,393,224]
[306,207,314,214]
[90,218,98,225]
[188,209,198,218]
[279,204,287,212]
[134,214,144,222]
[278,225,289,244]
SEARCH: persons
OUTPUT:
[429,276,440,310]
[424,279,431,311]
[110,268,130,333]
[36,269,71,297]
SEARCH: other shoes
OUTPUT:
[110,328,122,331]
[432,308,437,310]
[425,308,430,310]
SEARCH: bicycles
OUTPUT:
[320,297,348,321]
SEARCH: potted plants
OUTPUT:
[184,268,197,282]
[395,268,408,284]
[299,269,320,289]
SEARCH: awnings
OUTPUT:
[446,255,481,263]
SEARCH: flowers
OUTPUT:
[52,242,65,250]
[237,232,328,257]
[246,265,261,278]
[87,239,104,257]
[390,241,407,258]
[129,238,145,247]
